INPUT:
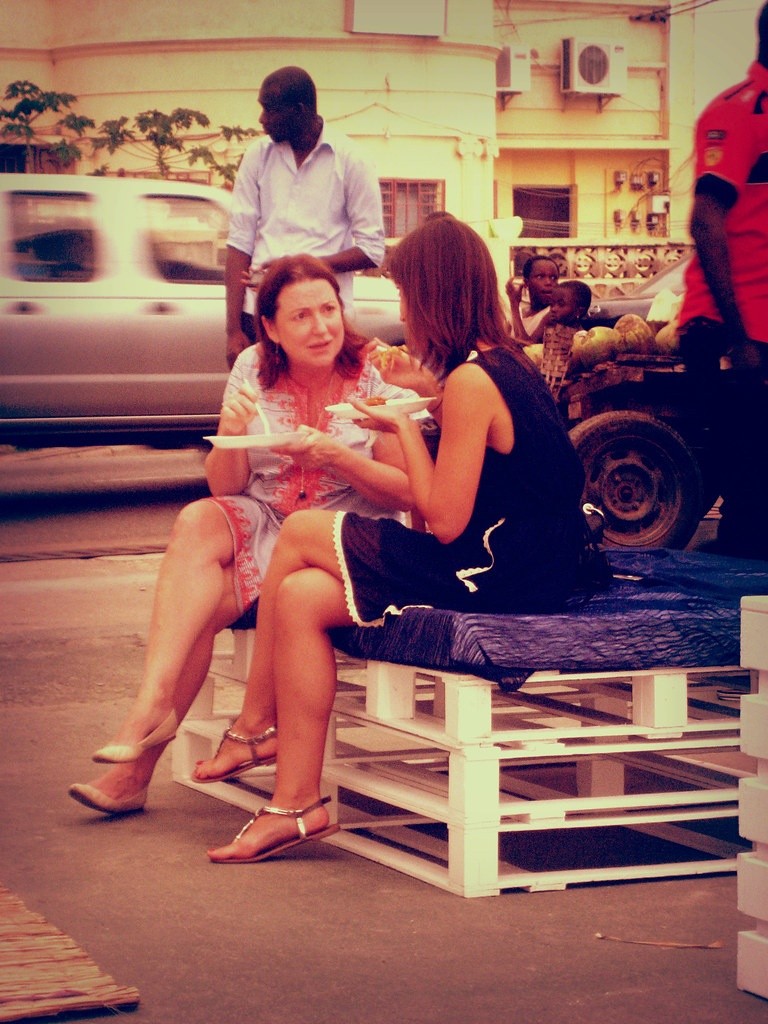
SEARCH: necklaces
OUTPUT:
[287,374,335,498]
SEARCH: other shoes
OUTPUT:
[92,708,179,764]
[67,782,149,813]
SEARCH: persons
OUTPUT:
[506,256,595,345]
[676,2,768,563]
[224,67,386,371]
[67,213,586,864]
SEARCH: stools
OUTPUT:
[171,544,768,900]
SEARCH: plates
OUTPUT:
[324,396,438,419]
[203,431,294,449]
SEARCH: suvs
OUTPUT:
[0,171,408,449]
[506,247,711,556]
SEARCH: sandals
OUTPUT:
[190,722,279,784]
[207,794,341,864]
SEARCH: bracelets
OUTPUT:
[428,398,442,412]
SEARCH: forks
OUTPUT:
[243,377,272,434]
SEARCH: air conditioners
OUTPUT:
[496,42,531,92]
[561,36,628,94]
